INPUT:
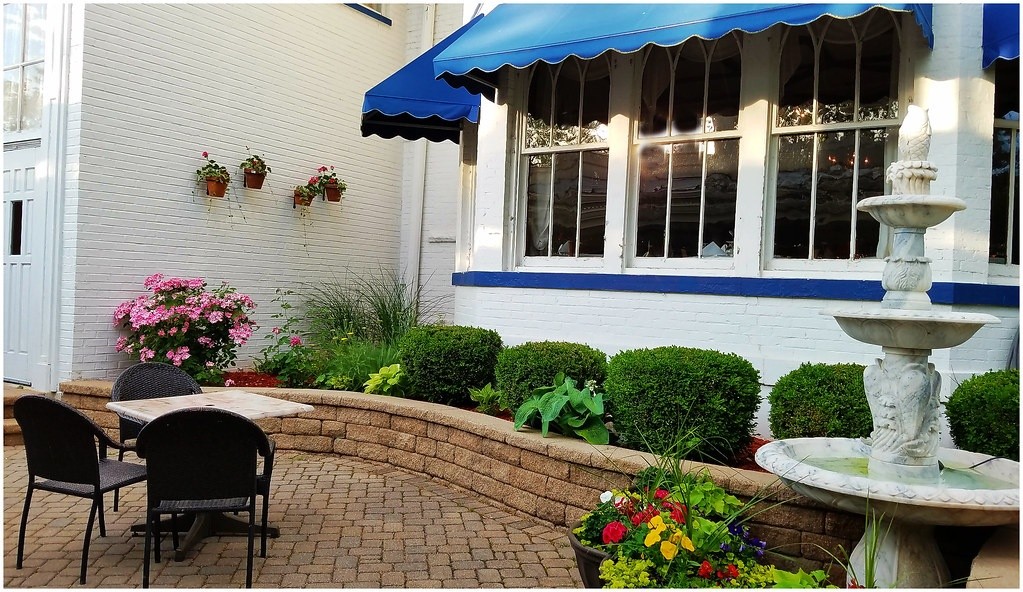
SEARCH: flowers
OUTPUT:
[583,419,899,589]
[239,146,270,169]
[191,151,231,179]
[308,165,342,184]
[112,272,259,386]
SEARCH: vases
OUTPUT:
[244,168,267,189]
[325,185,343,202]
[291,186,317,207]
[568,520,614,589]
[206,177,227,198]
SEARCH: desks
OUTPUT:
[106,390,315,561]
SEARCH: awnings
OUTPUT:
[361,12,484,144]
[432,4,911,103]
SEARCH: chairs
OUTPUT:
[13,394,179,585]
[112,363,203,510]
[136,406,276,588]
